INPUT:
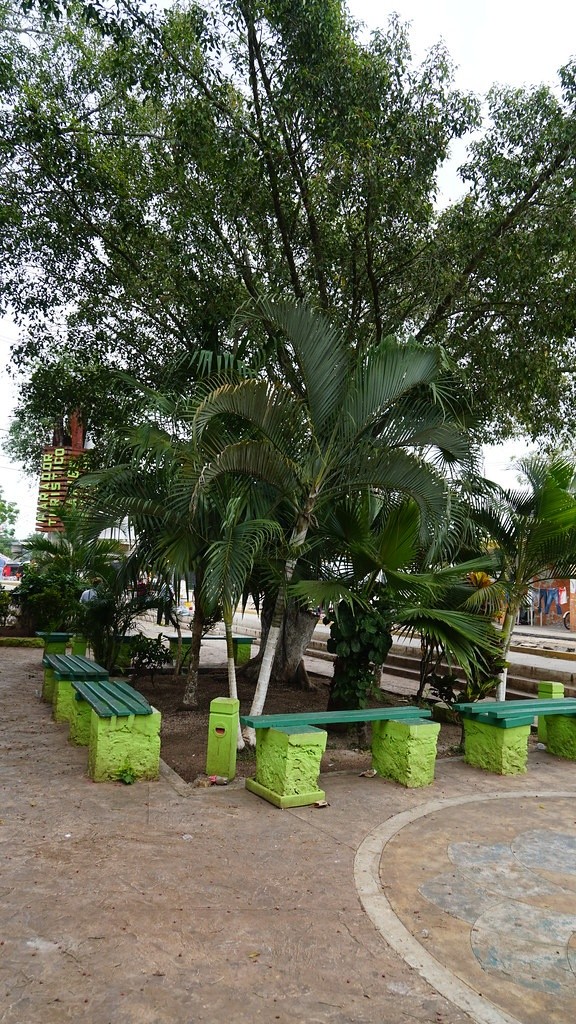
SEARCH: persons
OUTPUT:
[16,572,21,581]
[79,578,101,603]
[157,577,176,627]
[136,579,147,610]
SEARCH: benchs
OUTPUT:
[38,632,140,667]
[453,698,576,775]
[240,705,441,809]
[42,653,109,722]
[70,679,161,783]
[164,635,257,667]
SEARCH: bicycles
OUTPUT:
[563,611,570,630]
